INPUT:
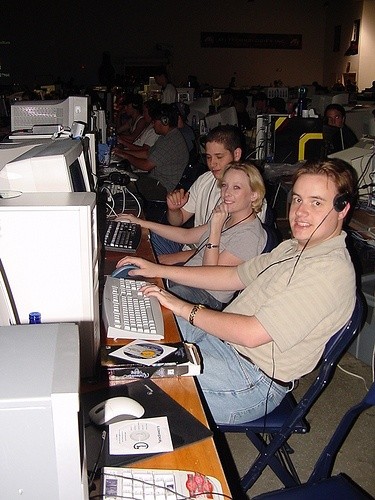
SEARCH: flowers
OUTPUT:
[273,79,282,85]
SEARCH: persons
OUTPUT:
[117,161,267,313]
[150,124,267,268]
[117,157,357,424]
[105,68,375,206]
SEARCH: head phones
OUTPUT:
[159,107,169,126]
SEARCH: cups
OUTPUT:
[98,144,111,167]
[302,110,308,117]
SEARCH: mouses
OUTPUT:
[112,264,140,279]
[89,397,145,428]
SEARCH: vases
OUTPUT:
[273,84,279,88]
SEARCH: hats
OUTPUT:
[118,94,144,105]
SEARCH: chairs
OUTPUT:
[135,139,375,499]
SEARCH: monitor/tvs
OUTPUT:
[1,97,97,327]
[186,98,239,133]
[311,91,375,203]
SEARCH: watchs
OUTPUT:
[189,304,206,327]
[206,243,219,249]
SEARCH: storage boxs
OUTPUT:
[345,273,375,367]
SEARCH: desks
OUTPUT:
[263,87,288,106]
[270,174,375,247]
[104,141,234,500]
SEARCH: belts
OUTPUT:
[219,338,292,387]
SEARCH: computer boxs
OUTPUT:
[0,109,107,500]
[91,91,113,122]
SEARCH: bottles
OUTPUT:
[29,312,41,324]
[107,127,117,148]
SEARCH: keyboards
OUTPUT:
[110,154,122,164]
[99,466,196,500]
[100,274,165,342]
[102,222,142,253]
